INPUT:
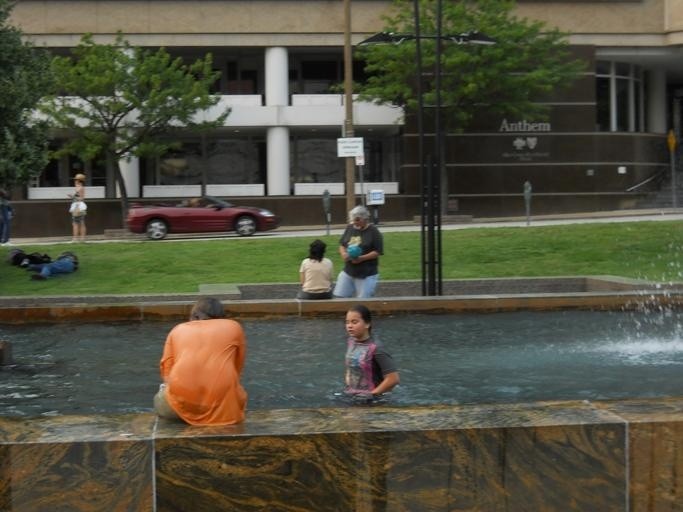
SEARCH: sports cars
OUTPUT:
[123,196,278,241]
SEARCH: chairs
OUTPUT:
[176,203,188,208]
[183,199,190,204]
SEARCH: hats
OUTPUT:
[74,174,85,180]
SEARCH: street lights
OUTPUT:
[354,29,497,296]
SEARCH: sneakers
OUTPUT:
[32,275,46,280]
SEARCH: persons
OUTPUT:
[154,299,248,427]
[190,199,214,209]
[344,306,400,396]
[296,240,334,299]
[8,249,51,266]
[0,188,15,243]
[66,174,87,243]
[26,252,79,280]
[333,205,384,298]
